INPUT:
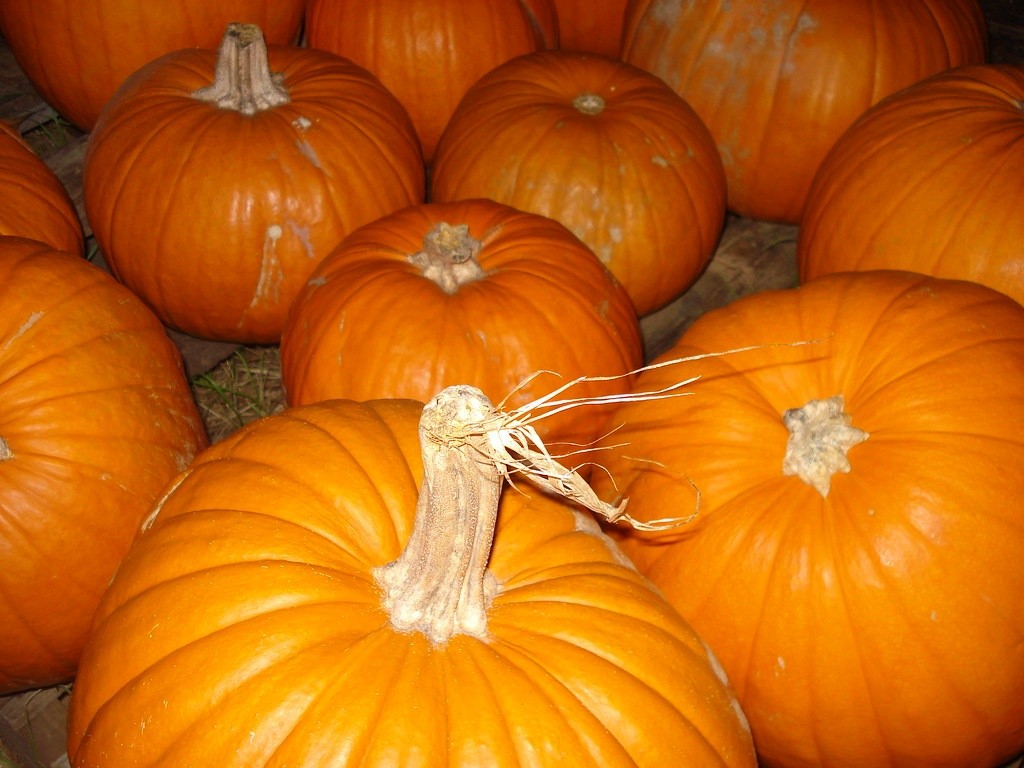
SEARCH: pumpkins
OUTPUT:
[0,0,1024,768]
